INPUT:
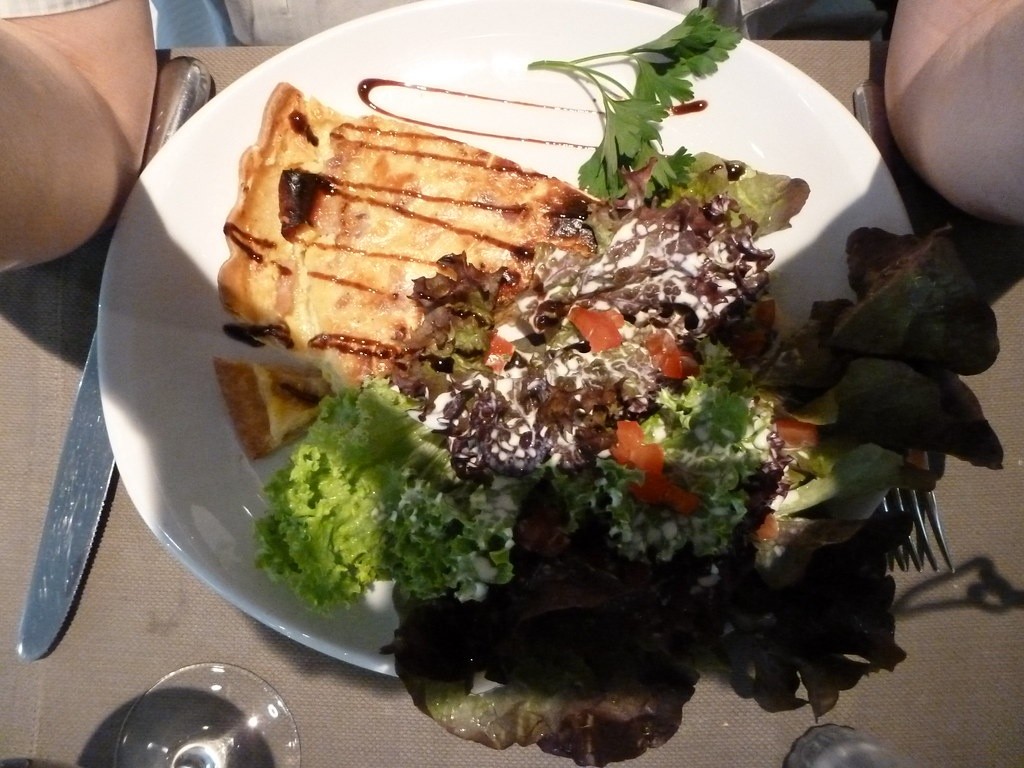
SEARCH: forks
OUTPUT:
[854,78,957,576]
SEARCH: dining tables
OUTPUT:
[0,42,1024,768]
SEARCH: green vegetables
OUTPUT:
[250,6,1024,768]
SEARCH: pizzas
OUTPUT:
[216,83,602,462]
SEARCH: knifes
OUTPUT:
[16,53,217,660]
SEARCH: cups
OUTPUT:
[110,663,301,768]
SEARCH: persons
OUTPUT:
[0,1,1024,266]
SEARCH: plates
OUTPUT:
[97,0,912,677]
